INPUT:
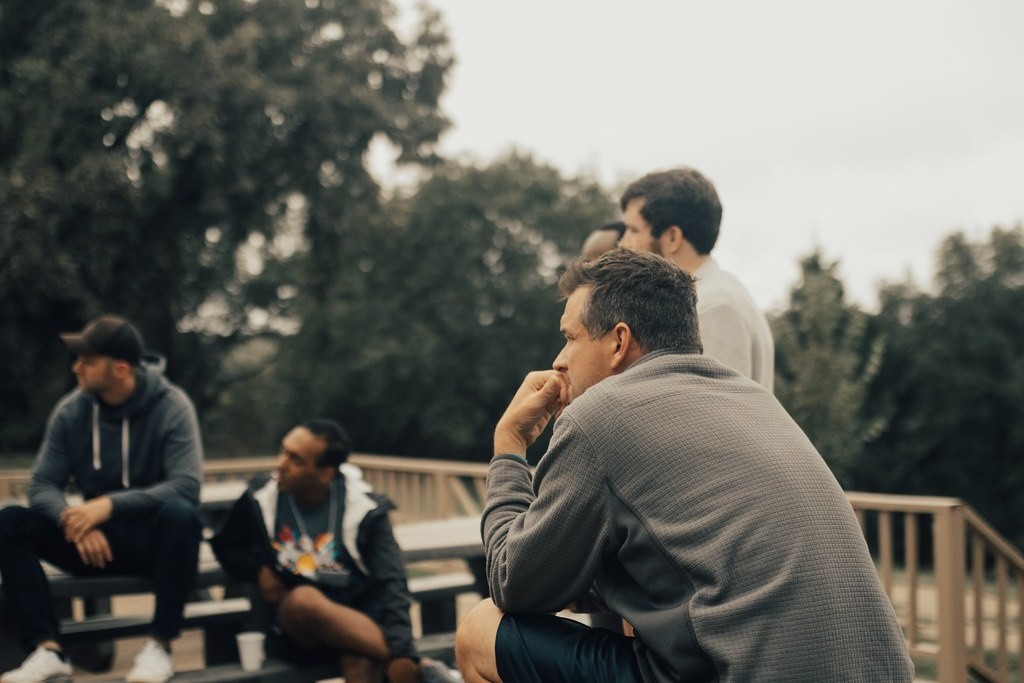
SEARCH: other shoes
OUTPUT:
[417,659,465,683]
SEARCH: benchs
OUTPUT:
[46,563,477,683]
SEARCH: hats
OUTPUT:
[61,317,144,365]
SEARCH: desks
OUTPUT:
[37,515,483,667]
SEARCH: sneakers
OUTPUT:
[0,646,74,683]
[125,638,175,683]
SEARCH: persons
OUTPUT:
[0,315,202,683]
[616,170,772,398]
[210,419,467,683]
[454,248,916,683]
[582,221,627,262]
